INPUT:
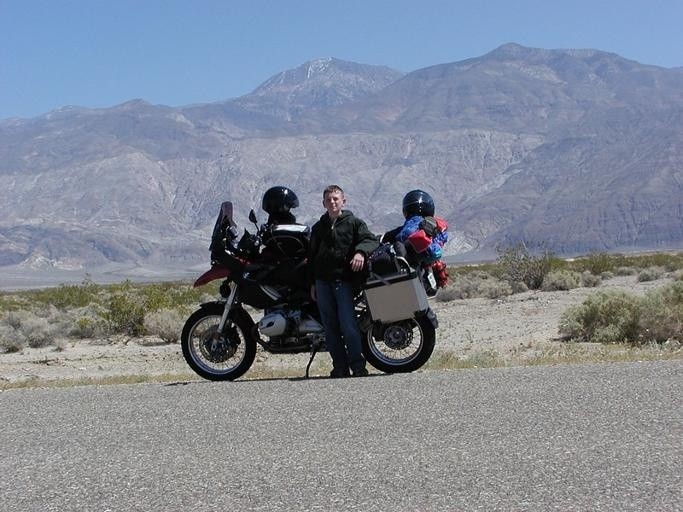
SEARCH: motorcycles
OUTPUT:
[180,200,442,380]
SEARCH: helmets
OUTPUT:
[402,190,436,221]
[261,185,300,214]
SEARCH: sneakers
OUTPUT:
[327,366,368,378]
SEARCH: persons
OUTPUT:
[307,184,381,380]
